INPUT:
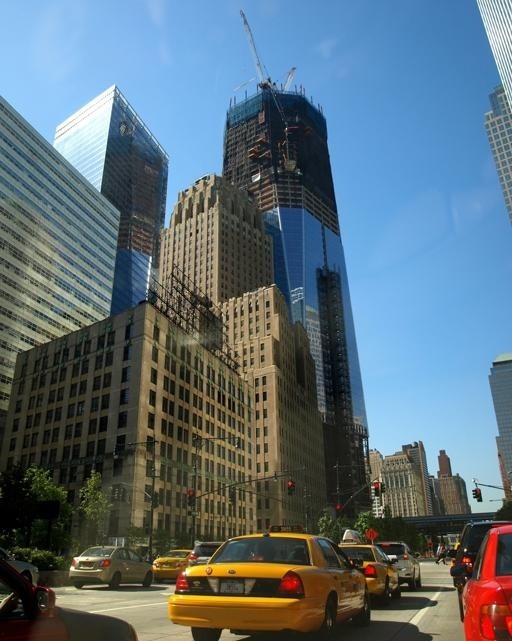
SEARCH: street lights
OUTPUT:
[114,435,159,564]
[334,463,372,503]
[273,469,308,534]
[192,435,240,550]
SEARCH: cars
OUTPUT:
[446,520,512,641]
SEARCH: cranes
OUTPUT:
[233,8,296,119]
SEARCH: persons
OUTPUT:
[450,551,474,624]
[435,542,447,565]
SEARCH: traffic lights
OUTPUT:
[374,480,385,497]
[188,488,195,506]
[288,481,296,496]
[472,488,481,499]
[228,492,235,506]
[336,504,342,511]
[152,491,159,508]
[111,487,128,501]
